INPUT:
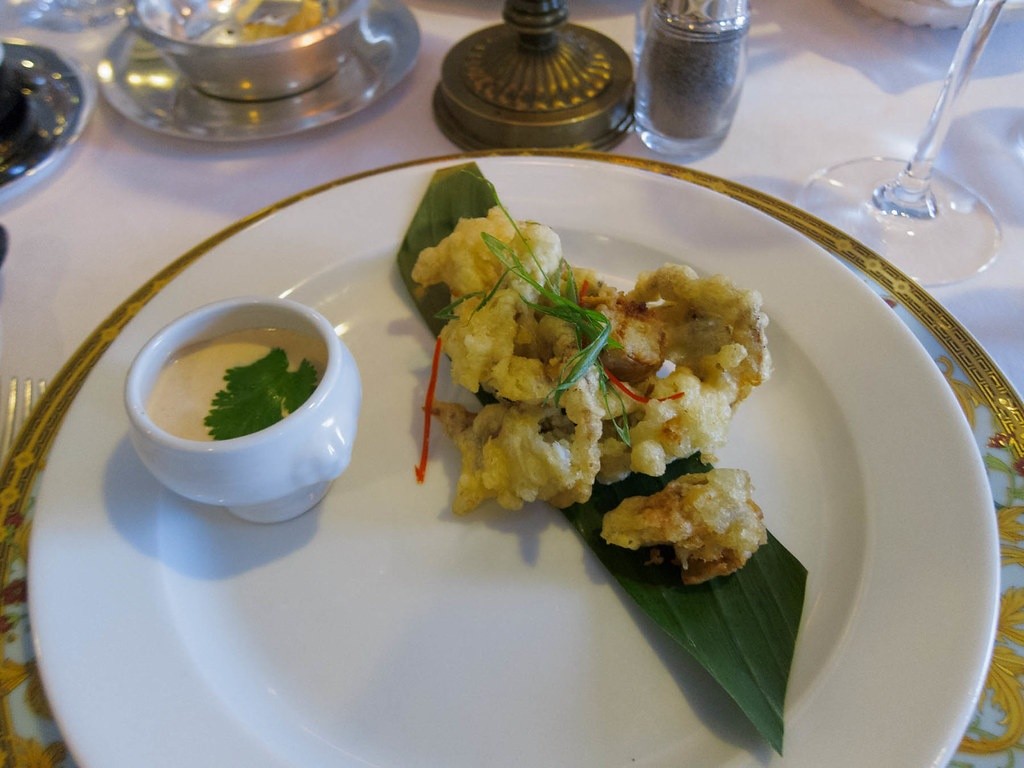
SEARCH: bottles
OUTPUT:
[633,0,747,159]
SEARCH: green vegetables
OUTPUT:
[202,349,317,440]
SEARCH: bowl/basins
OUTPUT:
[132,0,366,99]
[122,295,362,524]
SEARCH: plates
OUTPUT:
[0,36,92,204]
[0,150,1024,768]
[95,0,442,141]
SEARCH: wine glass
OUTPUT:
[798,1,1007,288]
